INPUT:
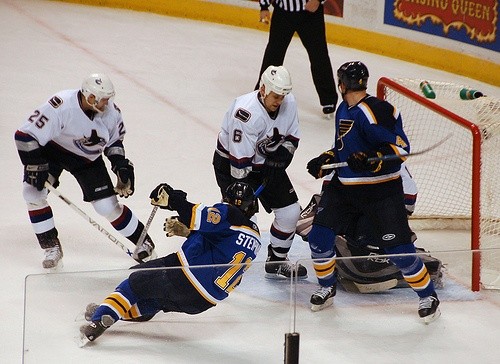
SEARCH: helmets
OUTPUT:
[260,65,292,95]
[80,73,116,102]
[338,61,369,91]
[224,182,258,220]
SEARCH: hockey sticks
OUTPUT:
[44,181,146,264]
[255,180,268,196]
[133,205,158,264]
[300,205,398,294]
[320,133,455,170]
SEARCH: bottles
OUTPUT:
[420,81,436,99]
[460,88,484,100]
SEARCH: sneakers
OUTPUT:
[264,244,308,279]
[42,243,64,273]
[75,302,100,324]
[311,283,336,312]
[138,235,157,263]
[417,290,441,325]
[323,104,336,120]
[79,314,115,348]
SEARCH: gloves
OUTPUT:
[24,160,49,191]
[347,152,384,173]
[149,184,187,211]
[112,158,134,198]
[163,216,190,237]
[306,150,336,179]
[263,145,293,180]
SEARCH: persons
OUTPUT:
[14,72,154,268]
[81,183,261,341]
[213,65,308,280]
[296,61,440,317]
[254,0,338,114]
